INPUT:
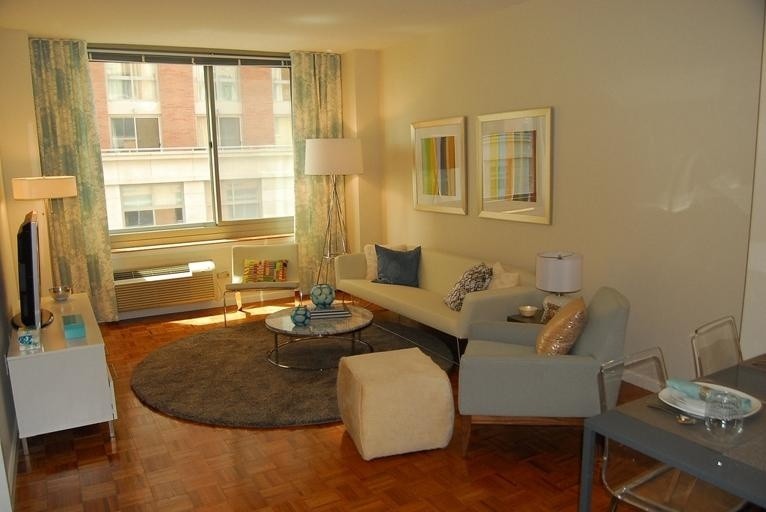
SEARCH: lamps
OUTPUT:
[10,171,81,215]
[533,245,587,316]
[301,136,368,300]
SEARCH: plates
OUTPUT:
[659,381,763,422]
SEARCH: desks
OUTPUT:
[572,348,766,512]
[506,308,543,323]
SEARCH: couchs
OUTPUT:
[332,243,553,363]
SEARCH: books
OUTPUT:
[310,302,352,320]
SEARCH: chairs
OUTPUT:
[217,241,305,332]
[455,284,634,461]
[593,341,752,511]
[686,316,746,374]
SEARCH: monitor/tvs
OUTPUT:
[12,210,54,330]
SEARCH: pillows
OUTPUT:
[442,260,495,313]
[484,257,521,290]
[361,242,408,284]
[239,256,289,285]
[369,241,422,289]
[533,293,590,360]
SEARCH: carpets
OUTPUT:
[126,316,456,431]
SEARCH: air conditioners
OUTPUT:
[108,259,219,317]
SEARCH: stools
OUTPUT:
[330,343,458,462]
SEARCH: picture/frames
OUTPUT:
[411,116,468,217]
[474,107,553,226]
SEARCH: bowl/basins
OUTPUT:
[518,305,538,317]
[47,287,72,300]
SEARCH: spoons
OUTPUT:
[647,403,705,425]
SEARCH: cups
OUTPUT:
[704,390,746,444]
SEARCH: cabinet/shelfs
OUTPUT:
[6,291,123,458]
